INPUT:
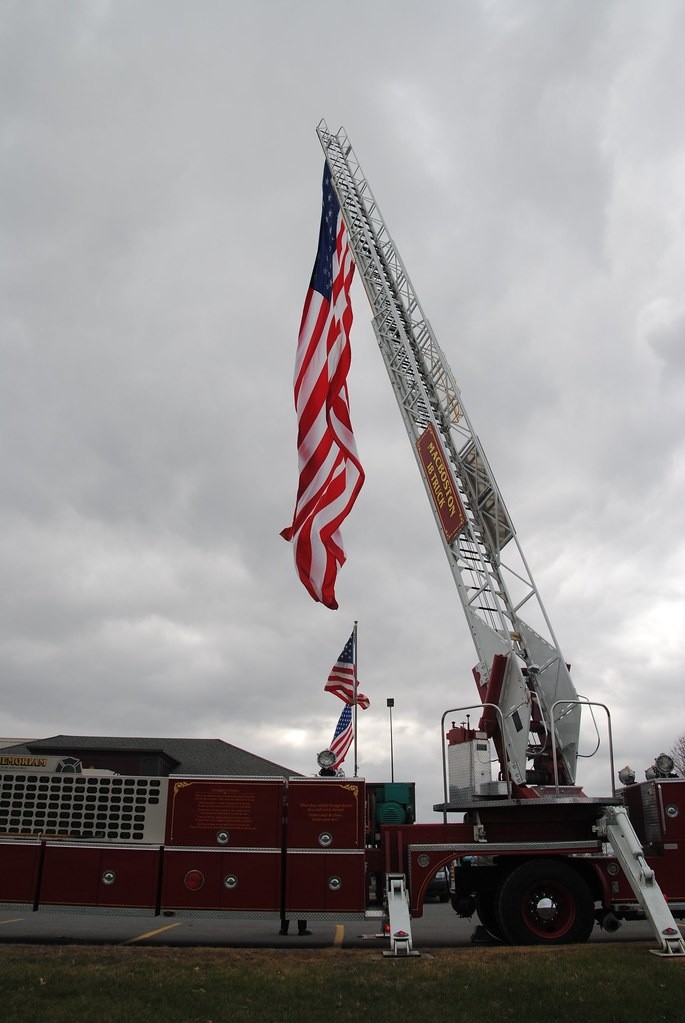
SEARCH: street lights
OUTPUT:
[387,698,394,783]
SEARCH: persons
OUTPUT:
[279,920,314,935]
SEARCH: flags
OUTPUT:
[280,159,365,611]
[324,631,370,710]
[330,705,353,770]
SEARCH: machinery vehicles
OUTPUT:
[0,123,685,957]
